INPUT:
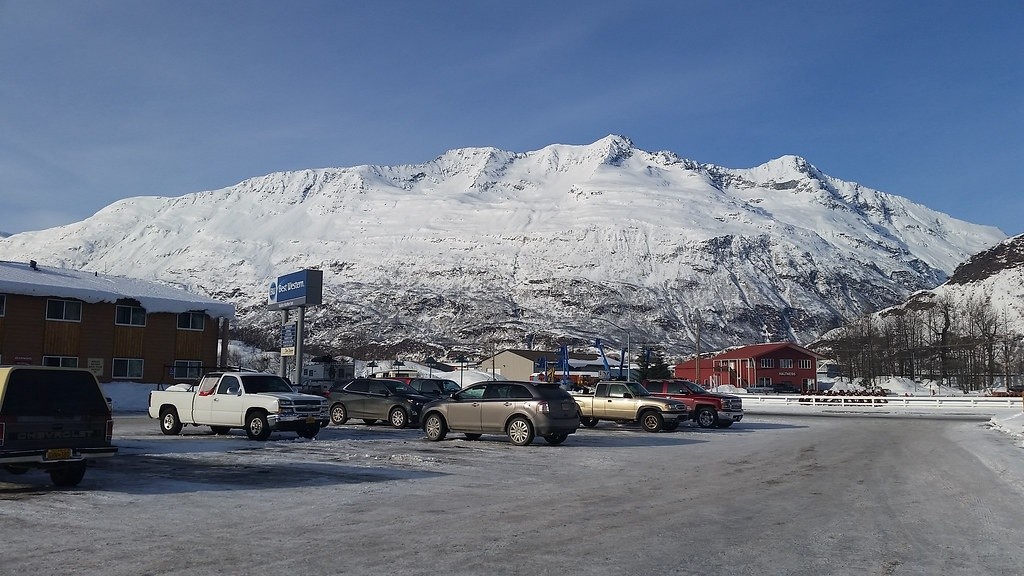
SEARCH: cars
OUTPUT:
[770,383,800,395]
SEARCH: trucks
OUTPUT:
[299,379,335,395]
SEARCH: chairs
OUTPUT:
[488,389,501,398]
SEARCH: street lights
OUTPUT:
[863,344,868,380]
[591,316,629,380]
[904,350,916,394]
[468,330,494,380]
[371,338,399,378]
[438,341,463,389]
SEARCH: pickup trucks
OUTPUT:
[642,379,744,429]
[566,381,689,433]
[148,370,330,442]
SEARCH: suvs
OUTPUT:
[385,377,478,399]
[325,378,437,429]
[0,364,119,492]
[418,381,584,445]
